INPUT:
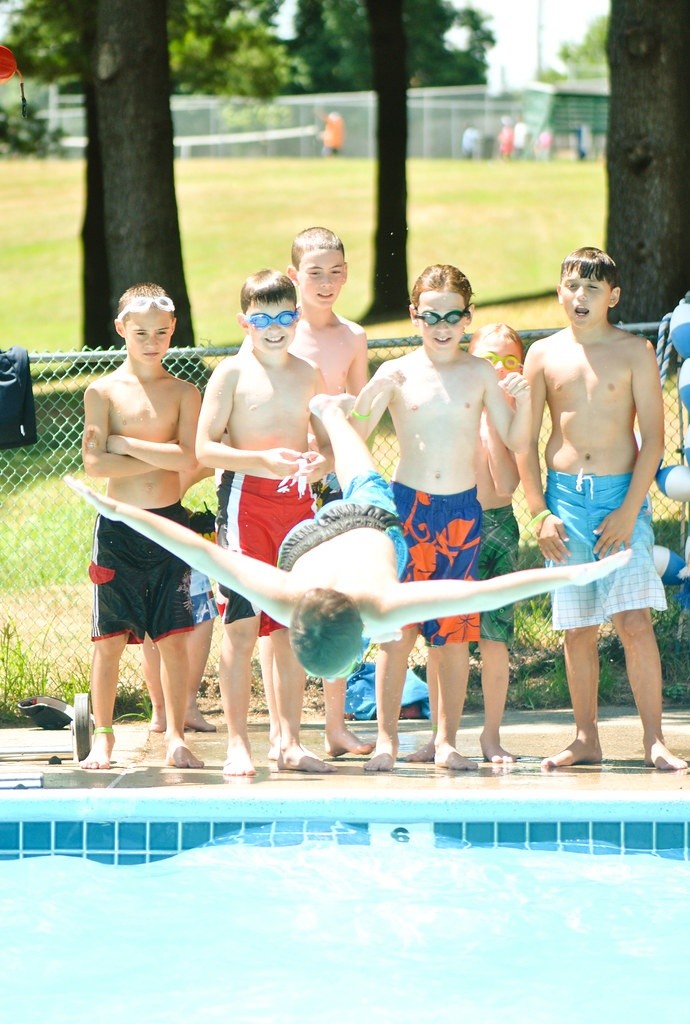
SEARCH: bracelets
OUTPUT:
[526,509,551,529]
[350,409,372,420]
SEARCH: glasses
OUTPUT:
[481,352,524,370]
[247,308,298,328]
[414,307,468,326]
[117,297,175,321]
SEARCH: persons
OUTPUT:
[67,225,688,772]
[460,114,592,161]
[315,110,346,156]
